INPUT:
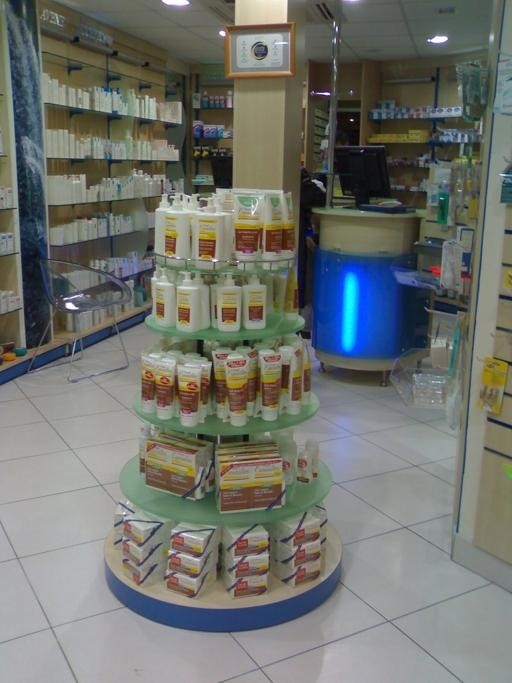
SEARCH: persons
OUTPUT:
[312,172,327,192]
[298,165,326,308]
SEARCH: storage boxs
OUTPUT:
[424,307,457,338]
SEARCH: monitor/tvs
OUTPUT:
[333,145,392,209]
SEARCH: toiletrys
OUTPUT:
[41,73,184,333]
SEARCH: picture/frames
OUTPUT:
[224,23,294,78]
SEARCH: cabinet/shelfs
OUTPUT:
[360,82,486,220]
[191,76,314,188]
[0,50,26,364]
[41,52,183,333]
[101,250,344,633]
